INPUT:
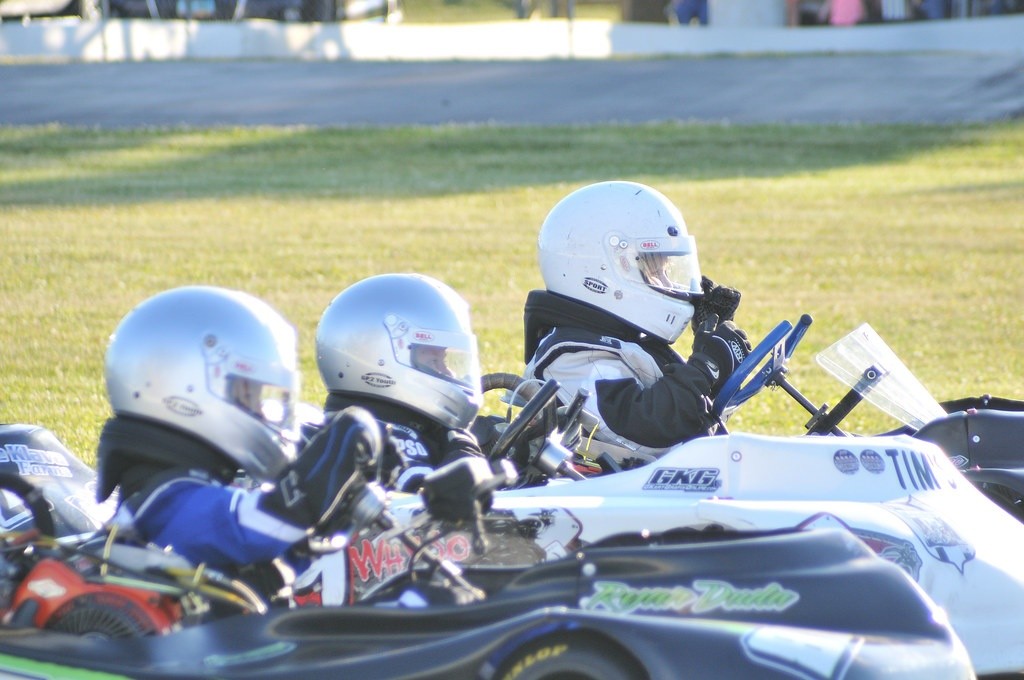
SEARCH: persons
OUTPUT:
[522,182,752,461]
[674,0,949,27]
[312,274,546,488]
[93,283,408,608]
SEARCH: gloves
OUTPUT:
[689,275,742,337]
[688,313,751,389]
[438,424,479,448]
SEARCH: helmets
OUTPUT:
[315,272,482,431]
[104,283,300,480]
[539,180,703,345]
